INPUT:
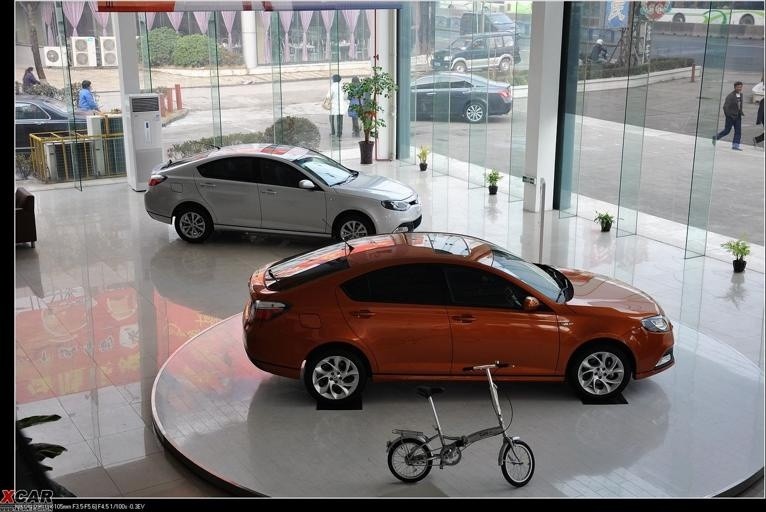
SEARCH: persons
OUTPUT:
[344,75,364,138]
[590,39,608,63]
[751,78,765,146]
[23,67,40,92]
[361,77,372,106]
[712,81,745,151]
[326,75,348,141]
[78,80,100,112]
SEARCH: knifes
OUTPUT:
[43,139,94,183]
[44,45,68,67]
[88,113,126,177]
[100,35,118,67]
[124,93,164,192]
[70,35,97,68]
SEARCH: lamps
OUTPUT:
[15,186,37,248]
[221,36,368,64]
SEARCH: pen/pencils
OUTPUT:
[459,11,522,43]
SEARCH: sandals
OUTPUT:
[656,1,765,26]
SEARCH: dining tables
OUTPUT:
[416,144,430,171]
[593,210,616,232]
[719,234,752,272]
[340,66,399,164]
[484,168,503,195]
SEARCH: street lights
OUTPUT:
[321,97,333,110]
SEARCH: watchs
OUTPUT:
[580,47,611,66]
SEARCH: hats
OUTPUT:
[15,92,90,153]
[238,229,684,409]
[137,134,431,248]
[410,71,515,127]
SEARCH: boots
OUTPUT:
[428,29,524,77]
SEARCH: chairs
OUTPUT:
[752,138,758,146]
[732,146,743,151]
[329,131,343,136]
[711,134,718,146]
